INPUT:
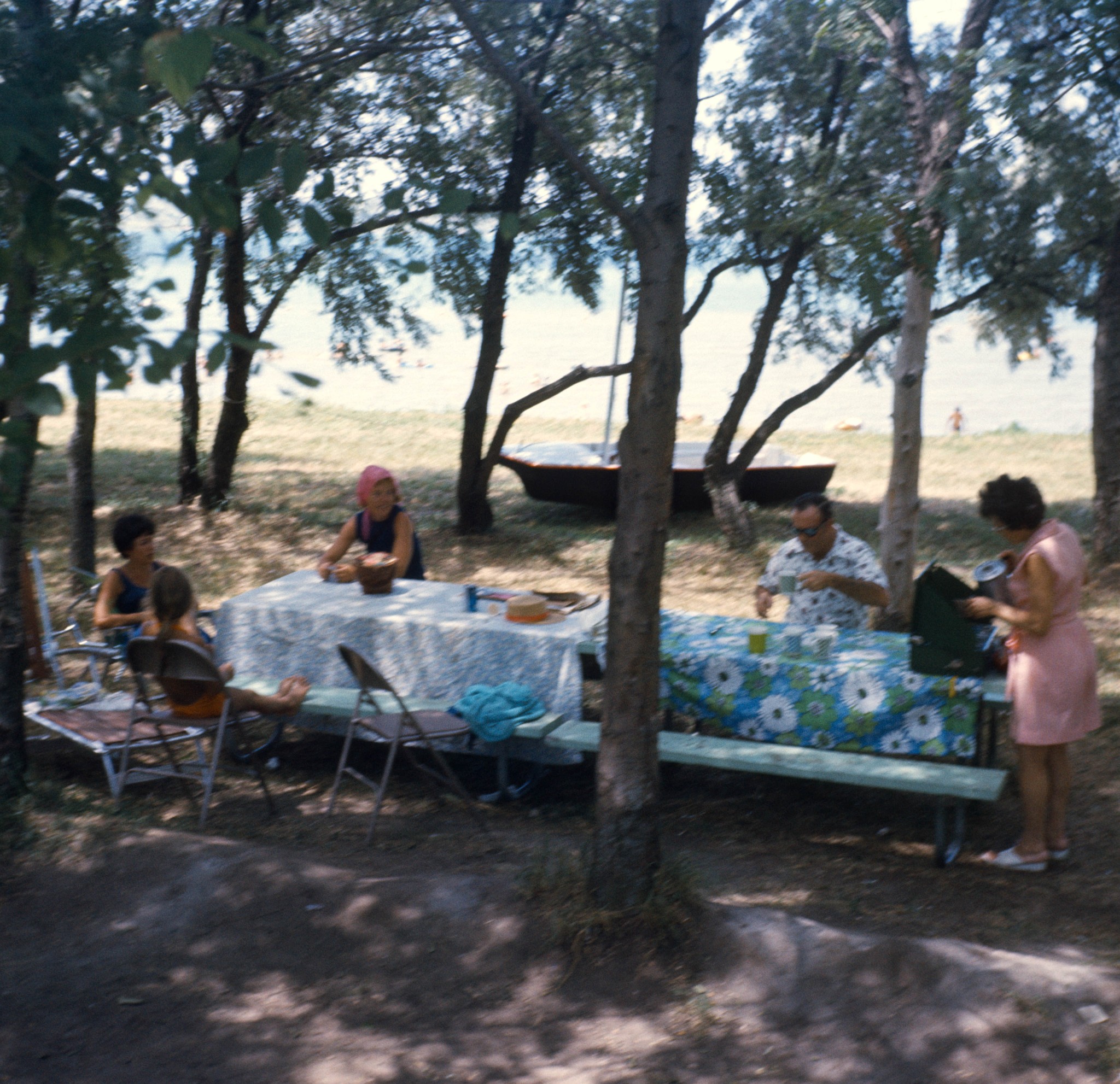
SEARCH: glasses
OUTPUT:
[792,519,829,538]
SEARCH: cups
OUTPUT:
[749,625,767,653]
[779,569,804,594]
[813,623,838,660]
[785,626,803,655]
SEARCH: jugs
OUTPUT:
[972,556,1010,602]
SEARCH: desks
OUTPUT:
[209,563,1018,871]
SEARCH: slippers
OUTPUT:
[1046,848,1069,861]
[979,847,1044,873]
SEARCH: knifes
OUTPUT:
[710,625,724,634]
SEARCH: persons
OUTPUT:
[752,491,891,633]
[946,408,963,435]
[318,464,423,583]
[93,513,308,720]
[957,473,1101,873]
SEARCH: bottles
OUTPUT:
[465,585,476,612]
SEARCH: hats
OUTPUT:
[504,594,565,625]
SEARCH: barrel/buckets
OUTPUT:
[352,551,399,593]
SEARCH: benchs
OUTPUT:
[543,717,1011,871]
[217,673,566,806]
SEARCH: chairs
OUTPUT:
[112,634,302,831]
[84,575,171,698]
[323,639,509,855]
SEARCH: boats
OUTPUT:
[499,440,840,514]
